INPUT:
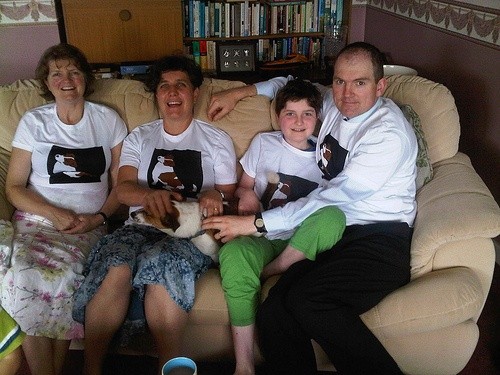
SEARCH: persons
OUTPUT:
[1,44,128,375]
[73,54,238,375]
[200,41,419,375]
[219,77,346,375]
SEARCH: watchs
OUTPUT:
[254,211,267,233]
[96,211,111,225]
[215,188,226,201]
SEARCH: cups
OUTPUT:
[162,357,197,375]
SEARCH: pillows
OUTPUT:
[382,74,461,163]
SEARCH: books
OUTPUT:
[182,0,344,37]
[183,37,323,71]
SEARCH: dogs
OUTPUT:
[130,197,253,264]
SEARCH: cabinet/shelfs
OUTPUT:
[181,0,353,87]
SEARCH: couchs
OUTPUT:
[0,74,500,375]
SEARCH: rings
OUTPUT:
[214,206,217,210]
[218,231,222,237]
